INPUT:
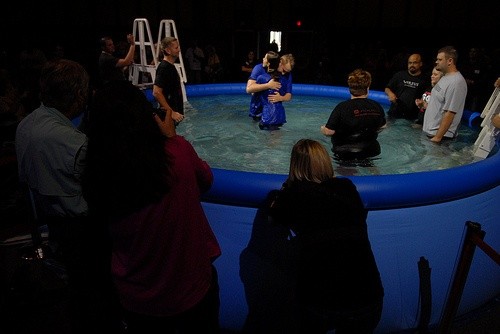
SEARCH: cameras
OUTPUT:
[155,108,166,121]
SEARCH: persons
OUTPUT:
[79,80,221,334]
[183,38,261,84]
[246,50,295,130]
[386,45,500,172]
[1,33,184,334]
[268,139,384,334]
[321,70,387,175]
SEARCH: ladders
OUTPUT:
[127,18,188,103]
[470,83,500,163]
